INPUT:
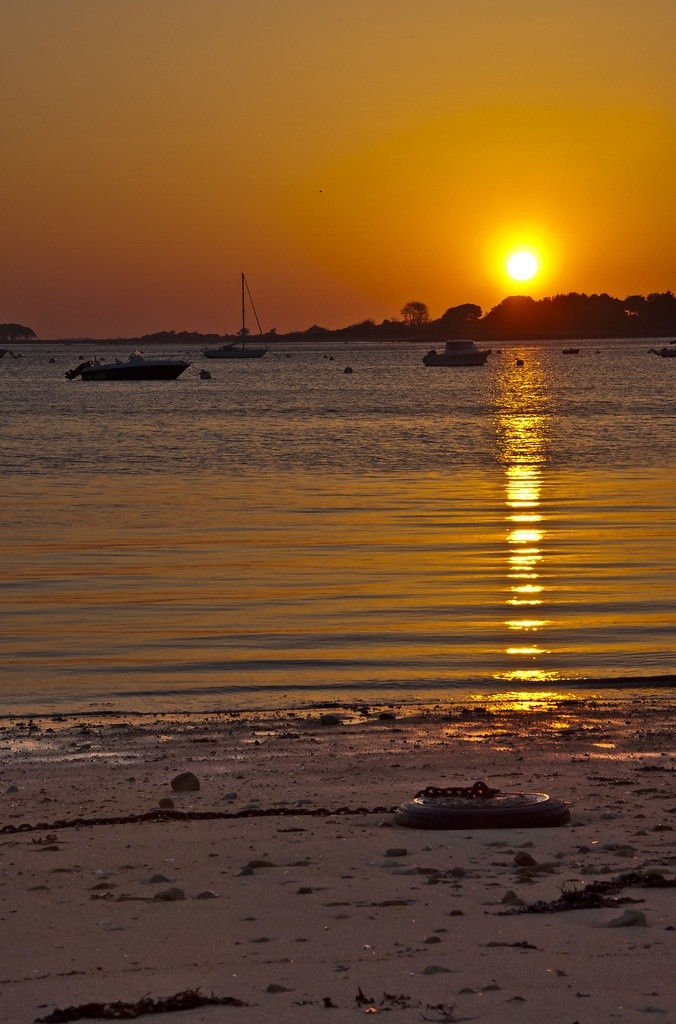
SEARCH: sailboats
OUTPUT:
[202,273,271,358]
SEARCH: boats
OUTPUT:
[422,340,493,367]
[66,351,192,381]
